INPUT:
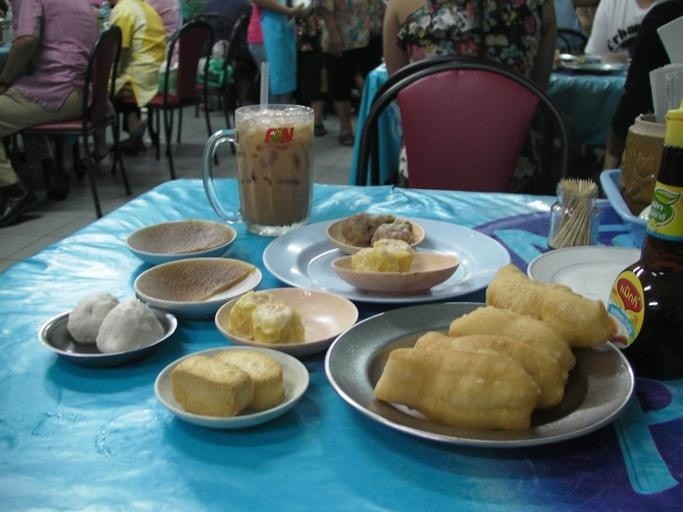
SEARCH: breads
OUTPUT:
[411,329,570,408]
[216,349,286,410]
[447,303,577,383]
[172,355,254,416]
[373,347,541,432]
[487,263,614,348]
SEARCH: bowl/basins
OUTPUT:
[126,219,238,267]
[327,217,425,255]
[38,307,178,367]
[215,288,359,358]
[154,346,310,430]
[330,253,460,296]
[132,258,263,318]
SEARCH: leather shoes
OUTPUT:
[0,184,40,227]
[44,170,72,201]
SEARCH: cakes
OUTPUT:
[373,238,416,273]
[350,246,400,274]
[250,301,304,345]
[227,290,273,340]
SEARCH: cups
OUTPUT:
[201,103,315,237]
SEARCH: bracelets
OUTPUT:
[0,79,13,88]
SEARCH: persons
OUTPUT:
[178,0,386,146]
[83,0,166,167]
[0,0,98,227]
[384,1,557,193]
[600,1,683,199]
[582,0,664,62]
[121,0,183,153]
[561,0,599,54]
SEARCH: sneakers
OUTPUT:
[336,130,356,148]
[110,132,149,158]
[312,122,329,137]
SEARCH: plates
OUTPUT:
[526,245,642,310]
[325,303,636,449]
[263,217,511,303]
[564,59,625,78]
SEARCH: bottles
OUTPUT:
[607,109,683,380]
[548,191,598,251]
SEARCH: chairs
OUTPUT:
[369,55,512,186]
[196,12,250,156]
[13,25,131,218]
[357,61,571,200]
[111,18,220,180]
